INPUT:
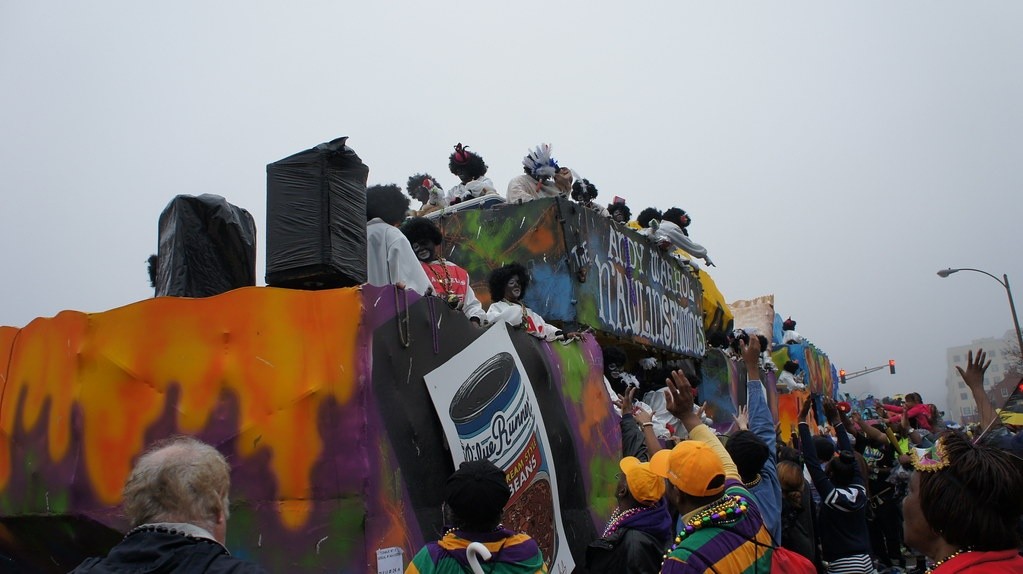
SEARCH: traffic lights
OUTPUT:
[889,360,896,374]
[840,370,846,384]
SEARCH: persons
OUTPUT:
[634,405,681,461]
[648,366,773,574]
[586,381,673,574]
[366,143,807,346]
[66,435,271,574]
[694,333,1023,574]
[404,460,552,573]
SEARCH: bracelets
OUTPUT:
[798,422,808,425]
[641,422,653,429]
[835,422,843,429]
[908,428,914,434]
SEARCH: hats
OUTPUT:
[650,440,726,497]
[620,456,666,506]
[726,430,770,473]
[447,458,511,520]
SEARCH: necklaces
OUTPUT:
[747,473,761,488]
[925,547,973,574]
[658,494,748,574]
[442,525,505,535]
[124,525,216,546]
[600,504,657,538]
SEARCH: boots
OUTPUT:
[907,555,926,574]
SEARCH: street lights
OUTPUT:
[937,267,1023,357]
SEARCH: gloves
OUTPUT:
[704,256,716,268]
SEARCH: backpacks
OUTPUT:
[674,504,818,574]
